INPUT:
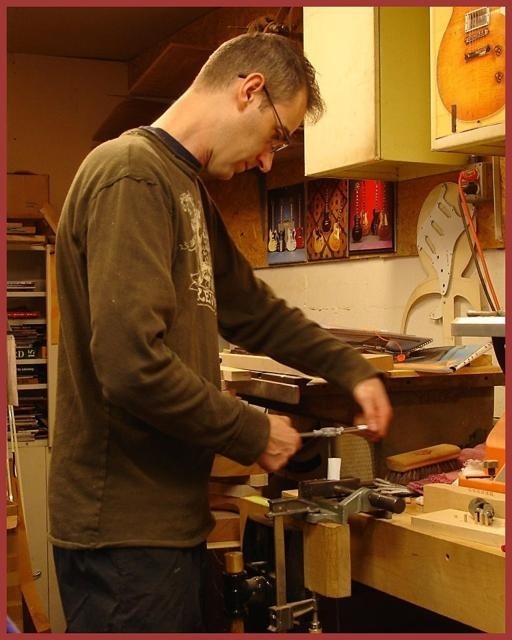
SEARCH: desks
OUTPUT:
[241,370,506,481]
[279,485,507,635]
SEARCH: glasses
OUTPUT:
[238,73,291,153]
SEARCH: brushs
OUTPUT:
[385,443,463,485]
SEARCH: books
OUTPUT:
[3,218,49,442]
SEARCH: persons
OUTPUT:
[44,30,395,632]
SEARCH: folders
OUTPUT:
[438,343,492,371]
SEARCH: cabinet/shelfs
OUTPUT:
[303,5,469,182]
[429,4,507,156]
[6,217,58,631]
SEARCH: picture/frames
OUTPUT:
[268,180,395,265]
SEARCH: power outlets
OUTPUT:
[457,162,492,203]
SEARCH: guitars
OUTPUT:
[352,180,392,242]
[311,188,343,254]
[437,5,506,121]
[268,198,305,253]
